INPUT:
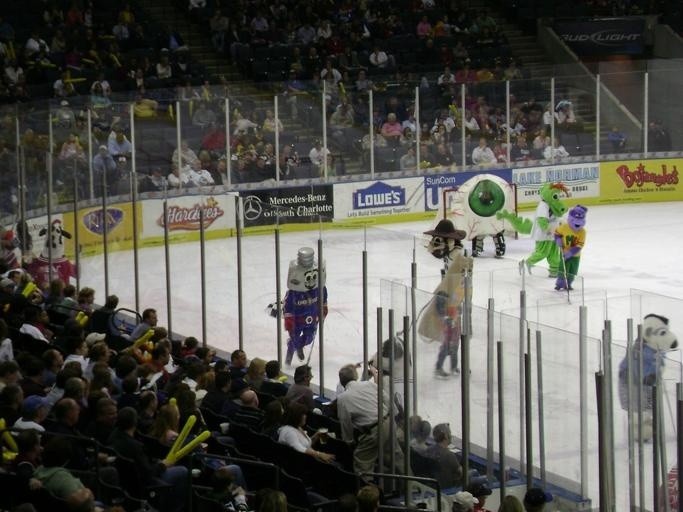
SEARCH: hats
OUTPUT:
[22,396,48,415]
[86,333,106,347]
[524,488,553,507]
[453,491,480,511]
[462,486,492,496]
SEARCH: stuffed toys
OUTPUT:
[449,174,515,260]
[614,312,680,440]
[496,182,570,279]
[553,204,589,294]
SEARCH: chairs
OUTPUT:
[0,1,597,217]
[0,279,455,512]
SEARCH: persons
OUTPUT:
[0,2,674,224]
[0,219,554,510]
